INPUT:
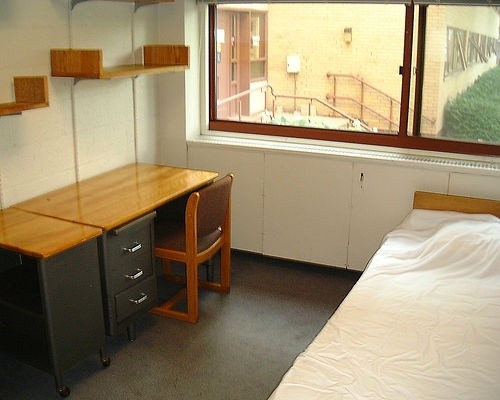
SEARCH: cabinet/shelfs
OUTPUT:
[0,0,191,117]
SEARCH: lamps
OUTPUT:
[344,27,352,45]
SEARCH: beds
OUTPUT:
[273,191,500,400]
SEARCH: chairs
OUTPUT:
[153,173,235,324]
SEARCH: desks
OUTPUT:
[0,207,112,399]
[11,162,221,343]
